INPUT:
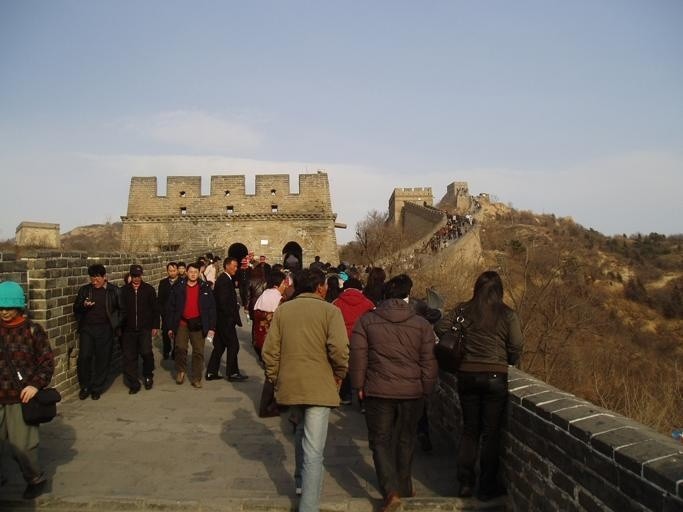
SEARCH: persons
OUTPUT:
[432,270,522,501]
[349,279,437,512]
[0,280,54,500]
[424,207,474,249]
[260,266,349,511]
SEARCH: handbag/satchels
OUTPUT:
[188,316,203,332]
[20,385,63,426]
[431,325,462,370]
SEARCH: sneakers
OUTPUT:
[294,486,305,497]
[79,352,250,400]
[415,435,435,451]
[338,397,367,415]
[478,484,507,501]
[458,481,472,499]
[21,476,51,498]
[402,483,418,499]
[260,409,281,418]
[375,492,401,512]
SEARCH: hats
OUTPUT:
[129,265,144,275]
[0,280,29,312]
